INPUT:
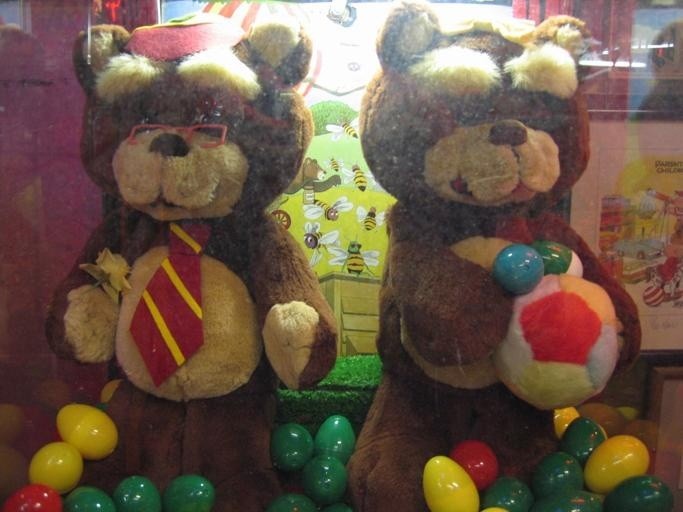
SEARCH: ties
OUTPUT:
[125,220,217,396]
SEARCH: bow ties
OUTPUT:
[491,211,566,243]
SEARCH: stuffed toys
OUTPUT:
[341,1,645,507]
[39,11,341,512]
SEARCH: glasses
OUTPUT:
[127,121,231,149]
